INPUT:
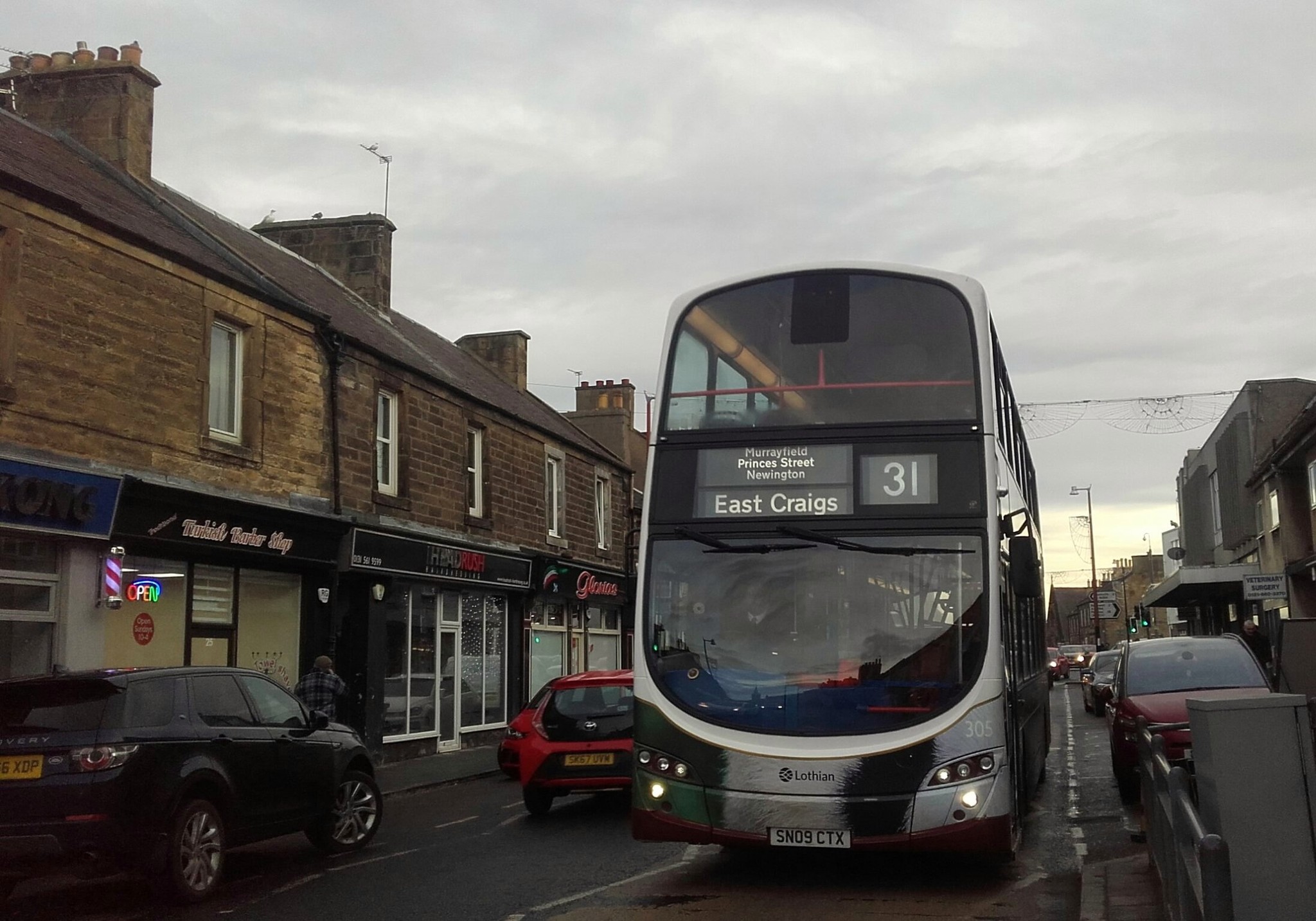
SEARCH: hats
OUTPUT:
[314,656,332,670]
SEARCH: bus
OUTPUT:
[623,261,1052,858]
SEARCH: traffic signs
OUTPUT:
[1090,603,1121,620]
[1088,591,1116,601]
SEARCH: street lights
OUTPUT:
[1143,533,1158,638]
[1070,484,1101,652]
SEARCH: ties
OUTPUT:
[751,617,757,631]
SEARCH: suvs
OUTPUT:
[0,665,382,905]
[1105,633,1277,793]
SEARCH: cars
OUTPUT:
[1047,644,1121,717]
[383,653,599,733]
[497,671,636,812]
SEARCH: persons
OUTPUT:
[293,656,346,723]
[1238,619,1272,676]
[719,577,790,647]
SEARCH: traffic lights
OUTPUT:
[1130,618,1138,634]
[1134,605,1151,628]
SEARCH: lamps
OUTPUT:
[97,546,125,609]
